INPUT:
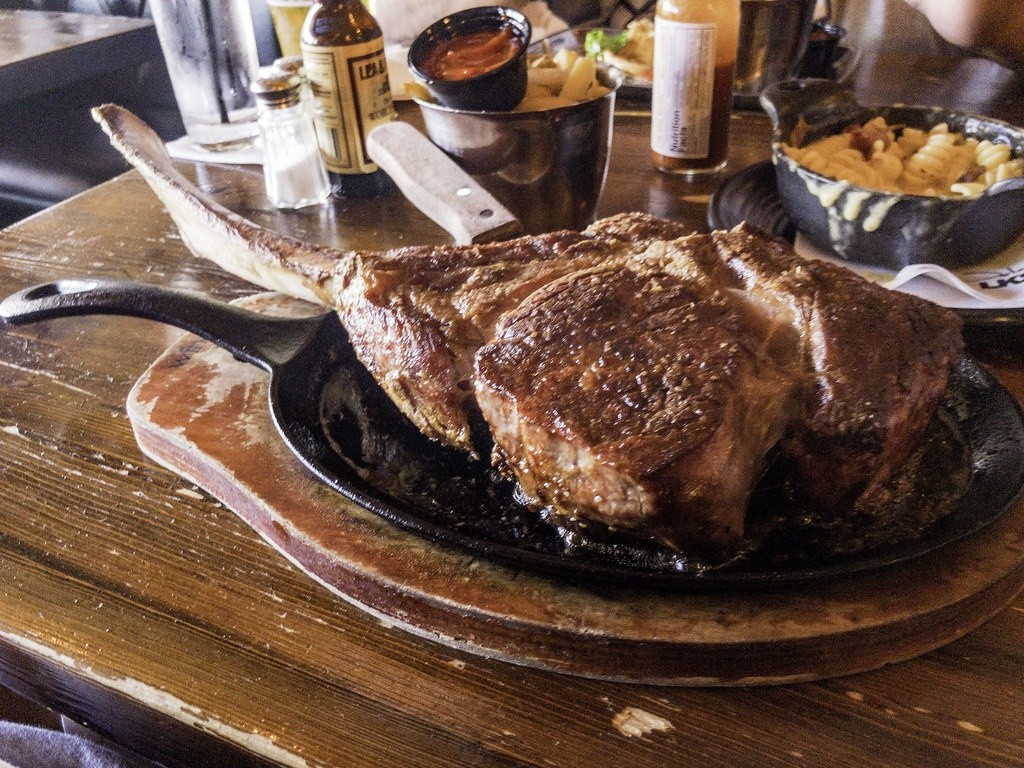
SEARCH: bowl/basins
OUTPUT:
[802,20,846,74]
[407,6,532,114]
[759,78,1023,271]
[736,2,817,86]
[409,56,625,237]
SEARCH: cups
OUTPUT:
[149,0,261,153]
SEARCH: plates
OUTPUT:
[708,156,1024,333]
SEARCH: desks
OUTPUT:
[0,9,160,108]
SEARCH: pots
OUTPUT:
[1,277,1023,595]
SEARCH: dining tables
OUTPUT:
[1,43,1024,768]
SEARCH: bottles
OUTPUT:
[650,0,740,178]
[246,65,333,212]
[301,1,398,201]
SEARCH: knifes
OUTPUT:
[367,121,530,249]
